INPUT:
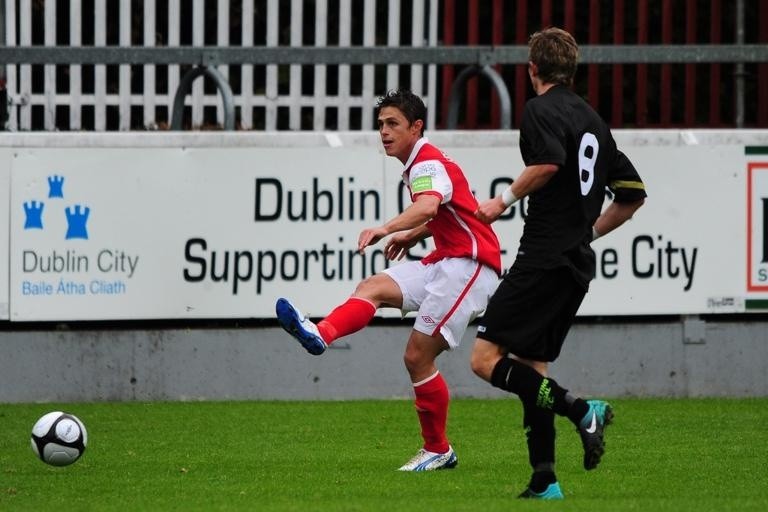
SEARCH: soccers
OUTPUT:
[31,411,88,466]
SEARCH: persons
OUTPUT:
[276,90,501,472]
[470,26,648,501]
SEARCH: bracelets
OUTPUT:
[502,186,517,207]
[592,225,600,240]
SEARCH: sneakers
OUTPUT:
[576,400,614,470]
[518,481,564,500]
[398,444,458,472]
[276,298,327,355]
